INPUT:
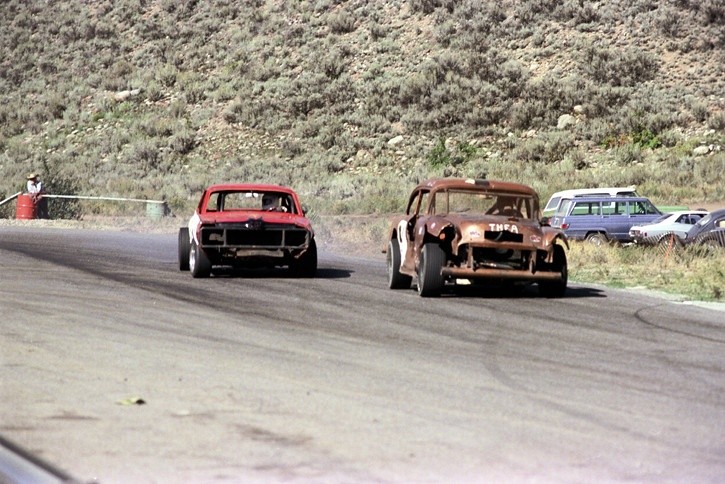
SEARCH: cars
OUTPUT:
[386,177,570,299]
[177,185,318,279]
[543,183,725,252]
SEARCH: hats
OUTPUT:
[27,174,38,180]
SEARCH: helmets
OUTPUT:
[262,194,279,209]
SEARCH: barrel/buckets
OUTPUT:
[15,194,38,219]
[147,204,166,220]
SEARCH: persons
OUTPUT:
[26,174,48,219]
